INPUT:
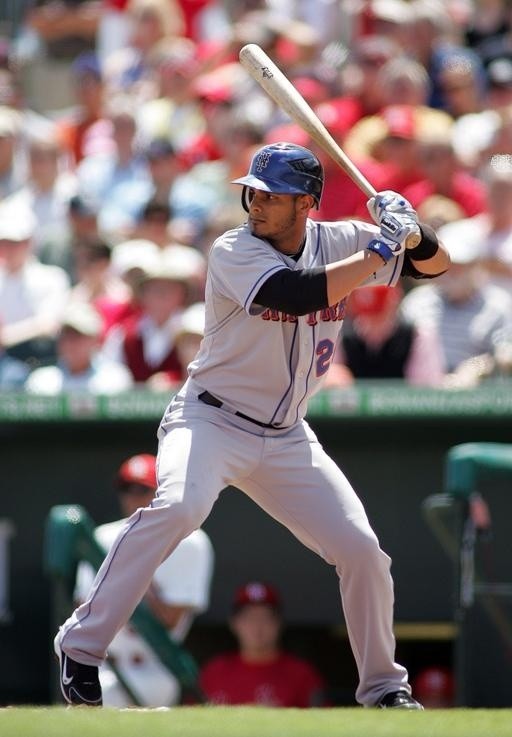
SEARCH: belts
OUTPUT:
[196,390,291,432]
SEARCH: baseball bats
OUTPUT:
[239,45,422,250]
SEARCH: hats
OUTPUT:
[110,454,161,492]
[233,580,279,608]
[229,141,325,210]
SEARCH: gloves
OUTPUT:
[366,186,418,266]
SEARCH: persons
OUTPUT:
[54,139,458,710]
[69,451,217,709]
[405,668,453,707]
[431,429,508,707]
[1,0,512,405]
[186,576,340,707]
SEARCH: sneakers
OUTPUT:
[51,629,104,707]
[376,690,423,712]
